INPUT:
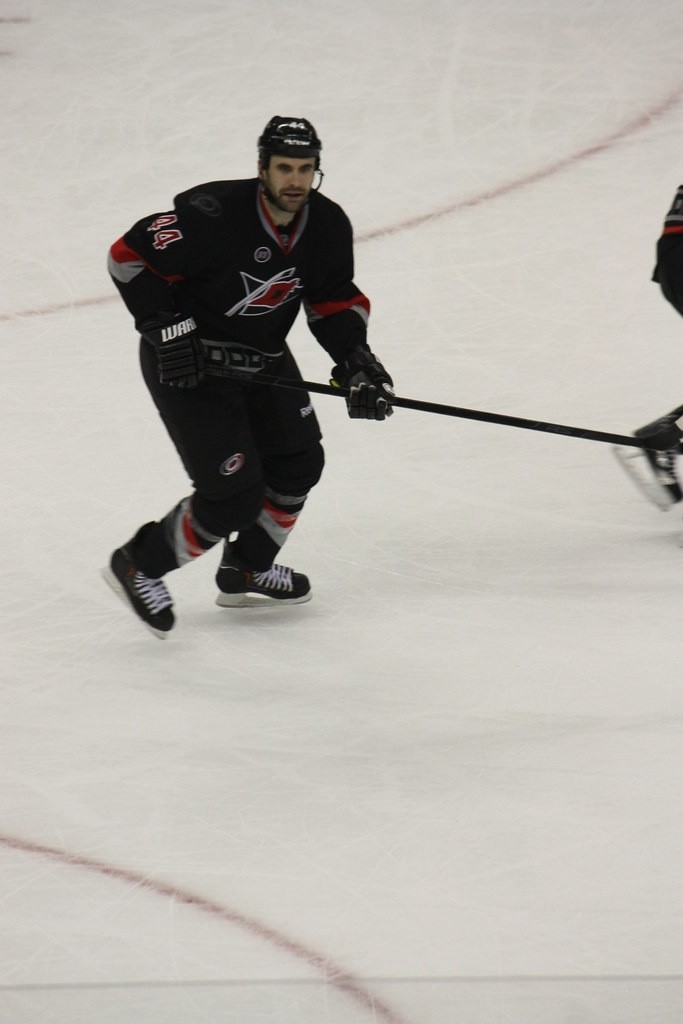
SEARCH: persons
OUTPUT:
[100,116,395,638]
[611,183,683,512]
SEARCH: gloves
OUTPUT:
[136,306,212,389]
[329,343,396,421]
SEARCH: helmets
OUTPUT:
[258,116,322,171]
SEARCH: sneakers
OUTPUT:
[101,521,174,640]
[610,414,683,513]
[215,531,312,608]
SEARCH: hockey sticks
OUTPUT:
[206,365,681,452]
